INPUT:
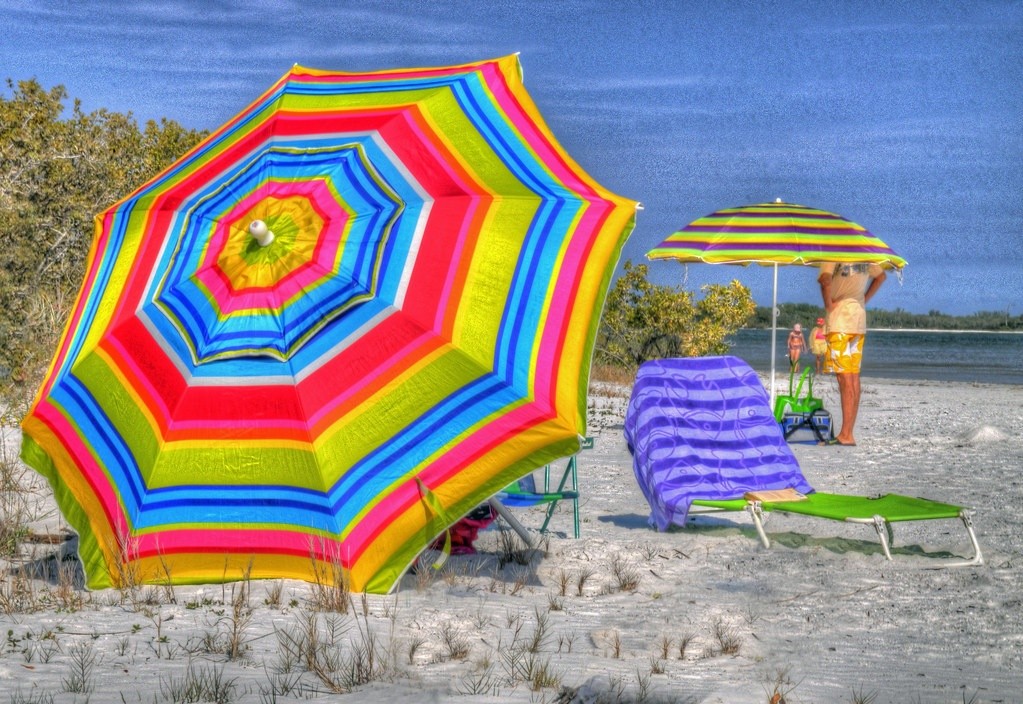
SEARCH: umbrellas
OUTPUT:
[646,196,908,409]
[20,51,645,596]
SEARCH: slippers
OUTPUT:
[817,437,857,446]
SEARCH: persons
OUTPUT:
[818,261,886,445]
[809,318,828,372]
[787,324,807,372]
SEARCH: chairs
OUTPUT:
[775,365,824,428]
[494,439,595,538]
[622,358,985,572]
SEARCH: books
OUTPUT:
[744,488,808,502]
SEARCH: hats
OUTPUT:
[793,324,801,332]
[817,318,825,325]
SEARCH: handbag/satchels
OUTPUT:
[428,507,499,555]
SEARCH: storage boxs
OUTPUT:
[783,410,833,445]
